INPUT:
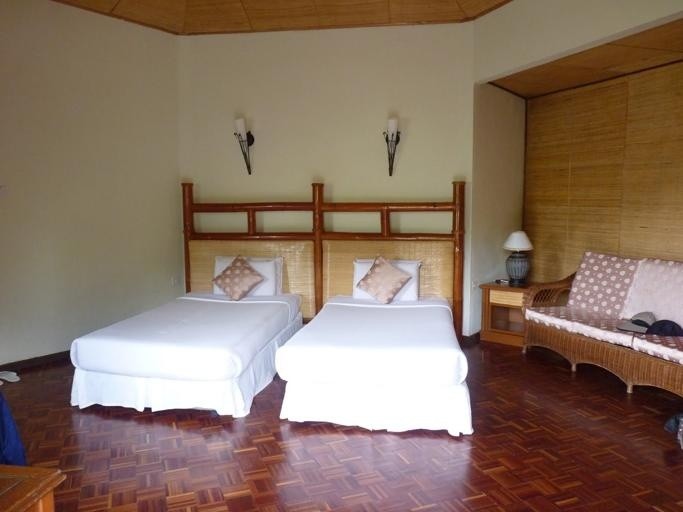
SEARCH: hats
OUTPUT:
[616,311,656,333]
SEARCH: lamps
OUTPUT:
[503,231,534,287]
[234,118,254,175]
[384,118,400,176]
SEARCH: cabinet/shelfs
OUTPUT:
[479,281,534,348]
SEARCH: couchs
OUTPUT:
[522,252,683,399]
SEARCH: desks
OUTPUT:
[0,464,67,512]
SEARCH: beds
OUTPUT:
[275,182,474,437]
[69,183,323,419]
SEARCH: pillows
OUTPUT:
[212,255,284,300]
[352,253,423,305]
